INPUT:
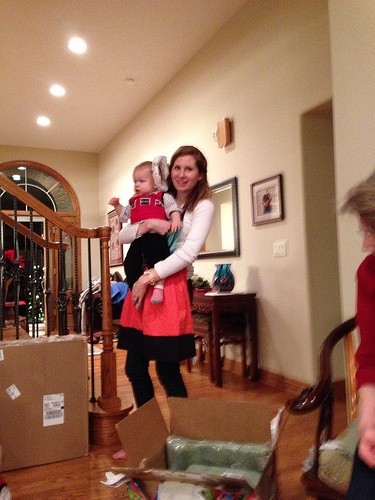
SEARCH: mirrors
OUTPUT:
[196,177,241,260]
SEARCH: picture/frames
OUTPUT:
[250,174,284,226]
[108,208,124,267]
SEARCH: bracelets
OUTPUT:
[144,271,155,286]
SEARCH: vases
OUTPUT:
[212,264,235,294]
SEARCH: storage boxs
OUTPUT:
[111,395,290,500]
[0,334,89,472]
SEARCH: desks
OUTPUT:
[191,292,258,387]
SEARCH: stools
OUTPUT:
[187,314,247,383]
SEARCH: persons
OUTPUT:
[341,170,375,500]
[109,161,182,304]
[116,145,215,410]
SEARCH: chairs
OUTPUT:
[285,314,361,500]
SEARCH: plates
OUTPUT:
[205,292,235,296]
